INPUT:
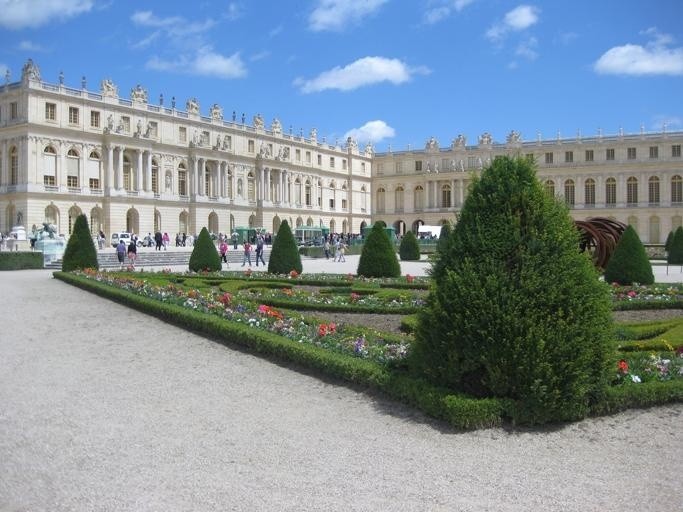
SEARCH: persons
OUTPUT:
[216,232,271,267]
[396,232,438,240]
[96,232,197,266]
[29,232,67,250]
[313,231,365,262]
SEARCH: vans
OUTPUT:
[110,232,143,248]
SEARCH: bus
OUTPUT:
[294,229,322,246]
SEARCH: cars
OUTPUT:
[143,236,156,247]
